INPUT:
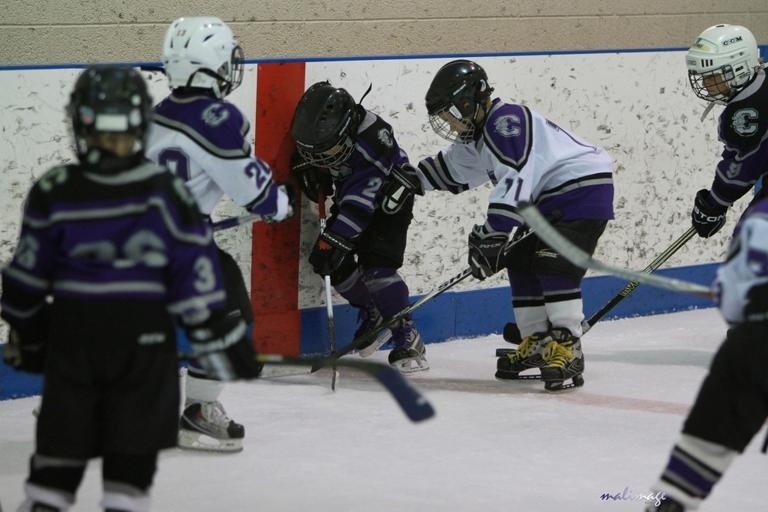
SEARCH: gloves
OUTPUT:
[691,185,729,241]
[307,227,357,276]
[372,161,425,227]
[467,224,509,283]
[713,209,767,329]
[292,163,339,207]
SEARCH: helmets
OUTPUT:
[65,59,152,139]
[288,80,363,170]
[685,22,764,105]
[423,58,496,145]
[162,14,243,94]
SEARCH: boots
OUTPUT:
[387,314,427,366]
[495,333,546,374]
[354,306,382,351]
[542,326,585,382]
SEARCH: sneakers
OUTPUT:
[180,395,246,441]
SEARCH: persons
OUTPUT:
[645,196,768,512]
[414,60,615,382]
[685,22,768,238]
[144,14,297,443]
[0,64,262,512]
[290,81,427,365]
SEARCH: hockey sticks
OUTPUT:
[504,226,698,346]
[520,200,723,303]
[176,353,433,421]
[256,210,565,380]
[319,195,338,391]
